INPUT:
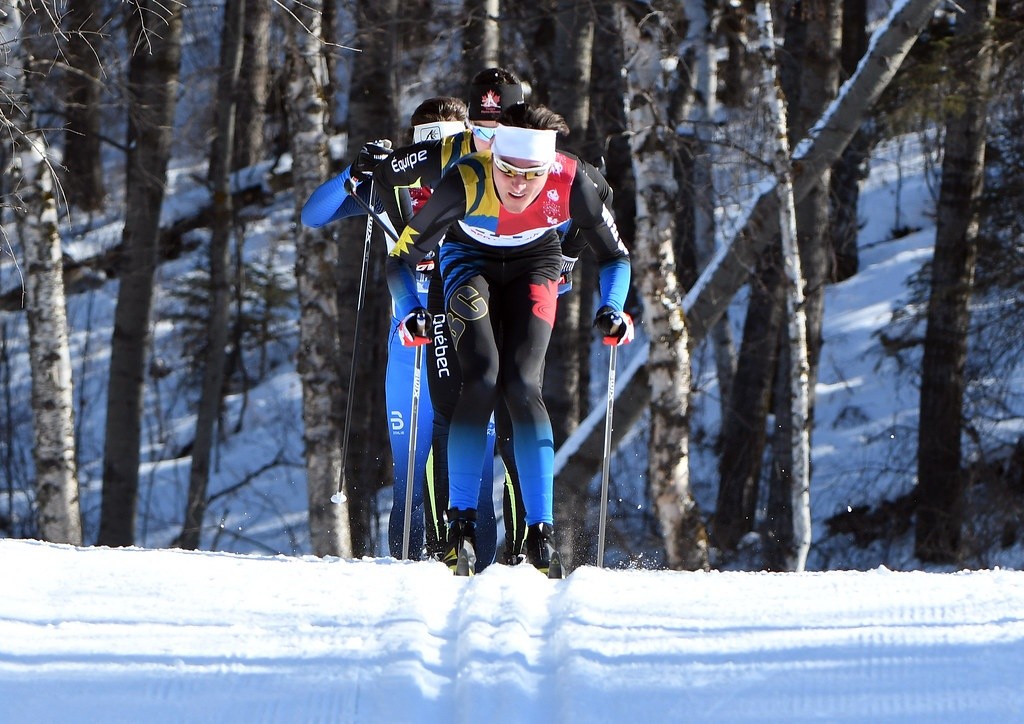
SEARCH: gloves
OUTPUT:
[592,311,634,346]
[416,249,435,276]
[350,139,393,181]
[557,270,573,298]
[399,308,433,346]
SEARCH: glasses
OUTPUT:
[493,153,550,180]
[465,119,497,142]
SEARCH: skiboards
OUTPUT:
[429,543,565,583]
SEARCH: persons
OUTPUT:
[384,103,632,576]
[300,97,498,575]
[373,69,615,566]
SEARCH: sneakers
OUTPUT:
[443,520,475,577]
[527,523,564,574]
